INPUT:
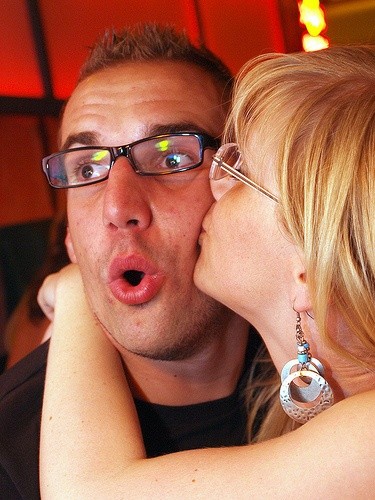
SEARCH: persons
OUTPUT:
[34,44,375,500]
[0,25,273,500]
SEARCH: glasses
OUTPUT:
[209,144,279,203]
[42,131,221,189]
